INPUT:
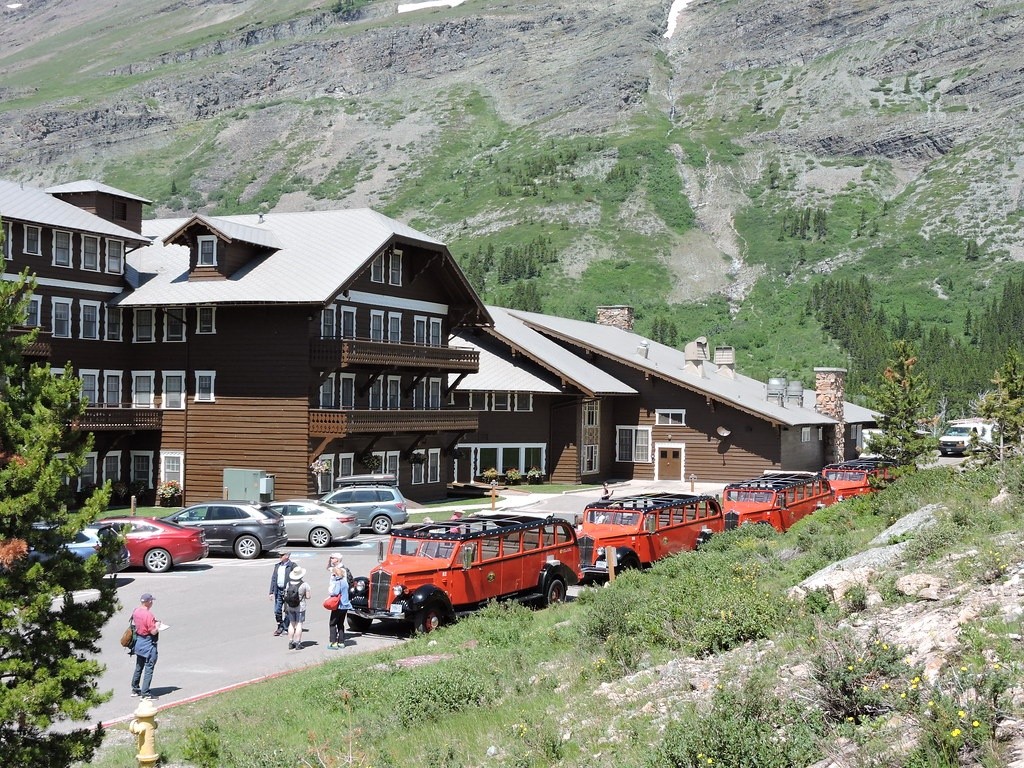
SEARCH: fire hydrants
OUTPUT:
[129,700,160,768]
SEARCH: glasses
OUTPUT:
[278,554,286,556]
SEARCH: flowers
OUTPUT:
[505,468,521,478]
[362,454,381,470]
[310,458,334,475]
[407,453,428,465]
[155,479,182,499]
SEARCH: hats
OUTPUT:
[333,567,345,577]
[140,593,157,602]
[289,567,306,580]
[278,549,291,555]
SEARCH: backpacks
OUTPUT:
[285,581,305,608]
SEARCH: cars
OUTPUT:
[94,515,209,574]
[267,498,361,548]
[28,521,131,579]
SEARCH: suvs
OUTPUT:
[318,473,410,536]
[160,500,289,561]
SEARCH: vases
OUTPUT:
[159,495,183,507]
[505,477,521,485]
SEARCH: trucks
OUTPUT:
[721,469,835,534]
[821,456,896,500]
[571,489,726,586]
[938,422,993,458]
[345,509,586,640]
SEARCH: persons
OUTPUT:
[269,549,299,636]
[121,594,162,701]
[327,553,355,593]
[327,568,346,649]
[285,567,310,650]
[599,482,614,499]
[450,509,466,534]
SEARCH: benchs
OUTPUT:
[421,532,566,565]
[735,485,827,501]
[601,509,714,529]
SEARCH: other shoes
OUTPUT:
[289,640,296,649]
[295,643,305,650]
[327,642,338,649]
[336,642,345,648]
[140,695,159,701]
[274,627,284,636]
[131,690,141,697]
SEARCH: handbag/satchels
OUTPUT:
[323,580,341,610]
[121,625,138,649]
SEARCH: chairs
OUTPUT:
[839,472,866,482]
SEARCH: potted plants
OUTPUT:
[484,469,499,484]
[528,471,544,485]
